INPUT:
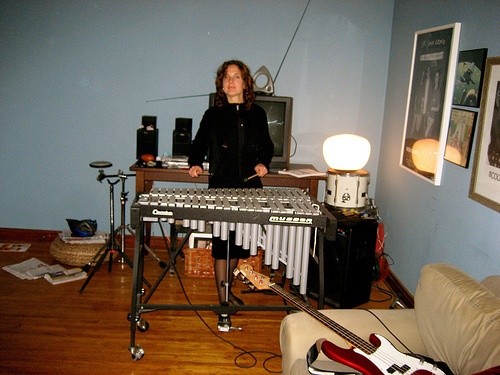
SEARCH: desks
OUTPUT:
[129,161,328,231]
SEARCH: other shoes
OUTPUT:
[217,314,232,332]
[227,291,245,308]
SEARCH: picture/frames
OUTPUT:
[451,48,490,108]
[443,108,479,169]
[468,55,500,214]
[398,22,461,187]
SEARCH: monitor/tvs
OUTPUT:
[208,93,293,172]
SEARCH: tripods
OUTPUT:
[79,170,166,294]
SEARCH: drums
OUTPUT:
[326,169,370,208]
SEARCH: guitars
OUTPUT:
[233,262,455,375]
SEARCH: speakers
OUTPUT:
[172,130,192,158]
[306,219,379,309]
[136,129,159,159]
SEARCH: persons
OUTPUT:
[188,60,274,322]
[410,61,444,140]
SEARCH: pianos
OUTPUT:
[127,188,338,362]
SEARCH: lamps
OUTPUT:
[322,134,371,172]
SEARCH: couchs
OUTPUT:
[280,264,500,375]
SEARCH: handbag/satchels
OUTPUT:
[66,218,97,238]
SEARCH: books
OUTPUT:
[43,267,88,285]
